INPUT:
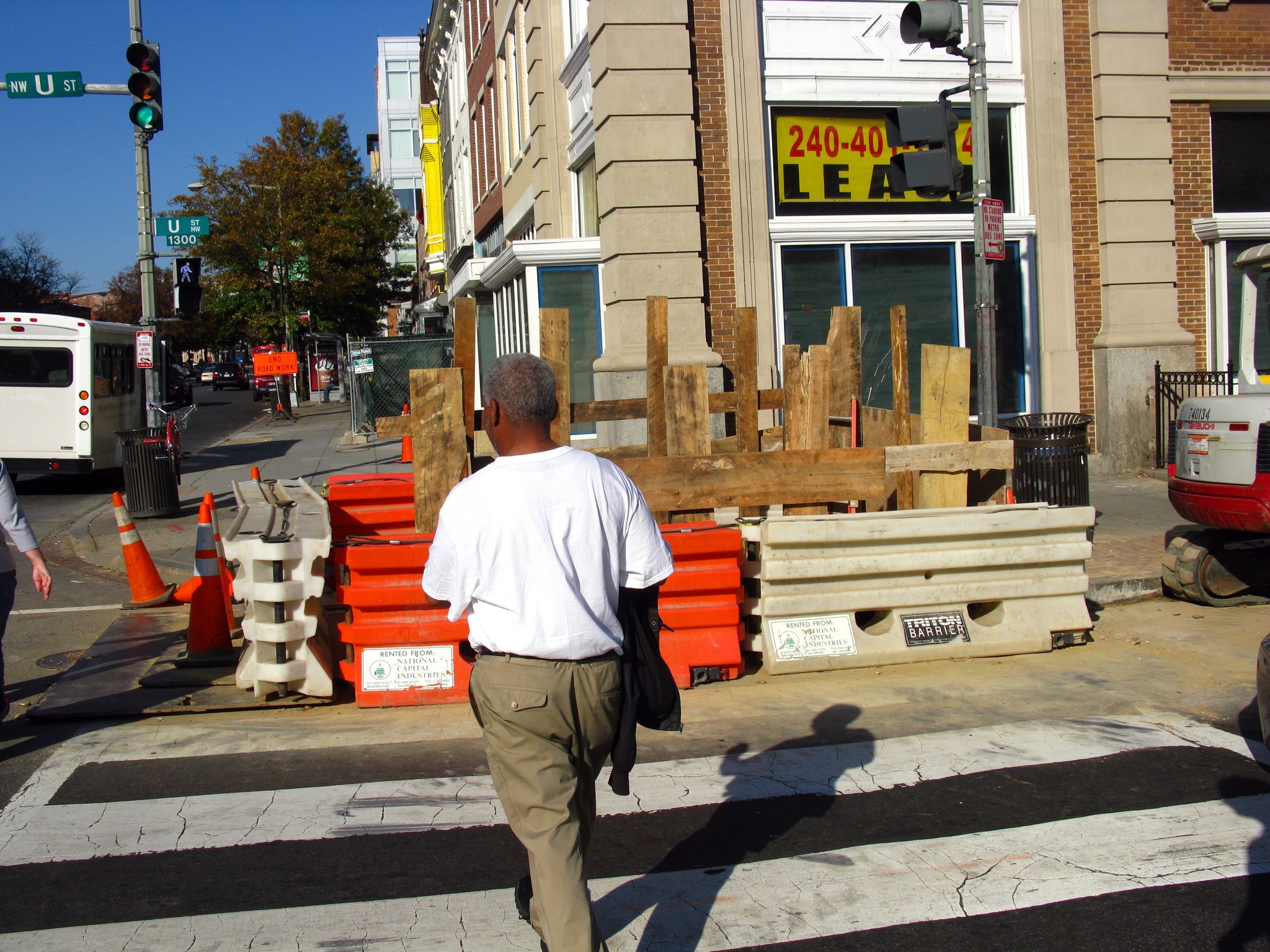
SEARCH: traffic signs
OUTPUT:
[982,198,1006,261]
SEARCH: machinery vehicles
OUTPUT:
[1160,240,1270,607]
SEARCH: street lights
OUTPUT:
[186,182,299,407]
[67,291,108,317]
[105,298,141,322]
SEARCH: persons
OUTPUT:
[419,353,672,952]
[321,364,340,404]
[0,458,52,722]
[186,359,211,367]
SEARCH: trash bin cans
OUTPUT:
[1007,412,1095,505]
[266,383,292,419]
[112,425,182,519]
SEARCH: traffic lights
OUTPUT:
[883,101,965,196]
[899,0,964,51]
[125,42,164,132]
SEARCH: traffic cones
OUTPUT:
[394,404,414,464]
[175,504,243,667]
[250,467,261,483]
[202,491,243,640]
[112,491,178,609]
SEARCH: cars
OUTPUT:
[252,375,290,402]
[201,367,216,386]
[167,364,193,411]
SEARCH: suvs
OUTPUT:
[212,361,250,391]
[195,362,218,384]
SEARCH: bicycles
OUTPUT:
[147,401,200,491]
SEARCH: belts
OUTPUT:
[458,639,522,657]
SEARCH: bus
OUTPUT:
[0,311,168,489]
[251,344,287,376]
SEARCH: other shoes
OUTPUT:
[514,873,550,952]
